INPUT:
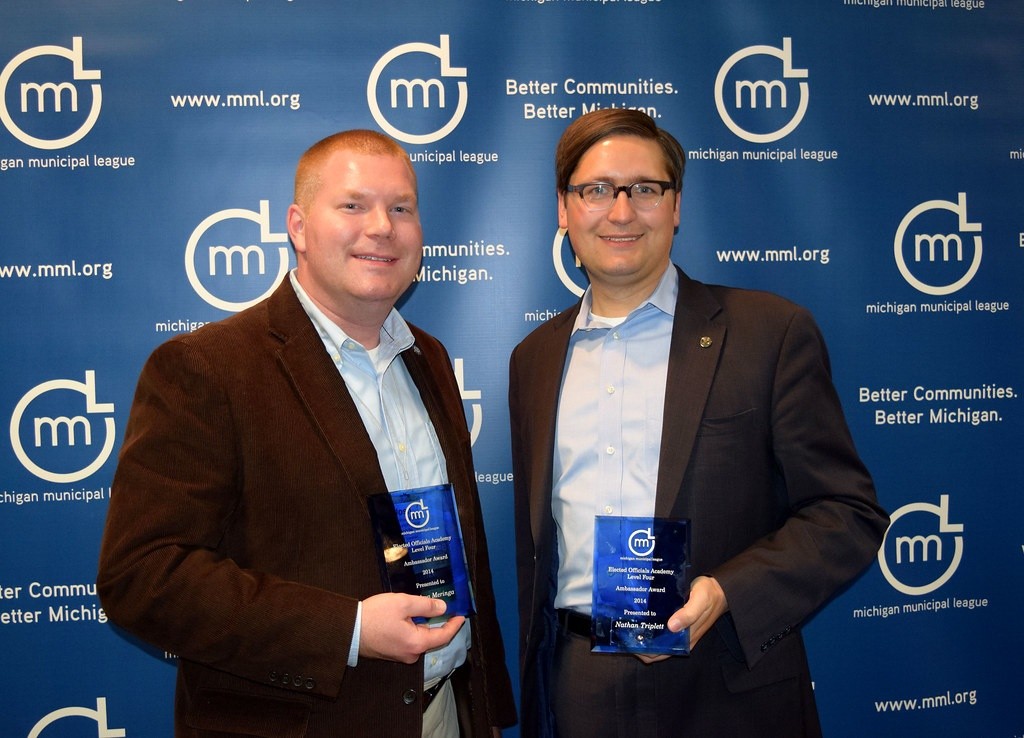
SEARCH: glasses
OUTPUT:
[565,179,675,212]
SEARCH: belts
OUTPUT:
[423,674,449,713]
[558,609,592,638]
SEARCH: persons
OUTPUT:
[507,109,893,738]
[98,131,518,738]
